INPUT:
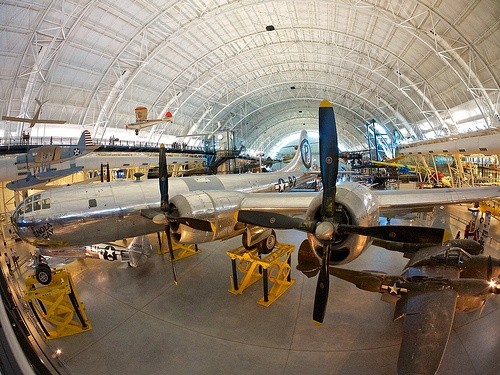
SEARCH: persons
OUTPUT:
[372,169,375,175]
[10,248,19,268]
[171,140,188,150]
[4,253,13,276]
[386,217,391,225]
[453,219,486,255]
[22,129,26,144]
[108,136,112,145]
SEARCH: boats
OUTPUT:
[2,99,499,375]
